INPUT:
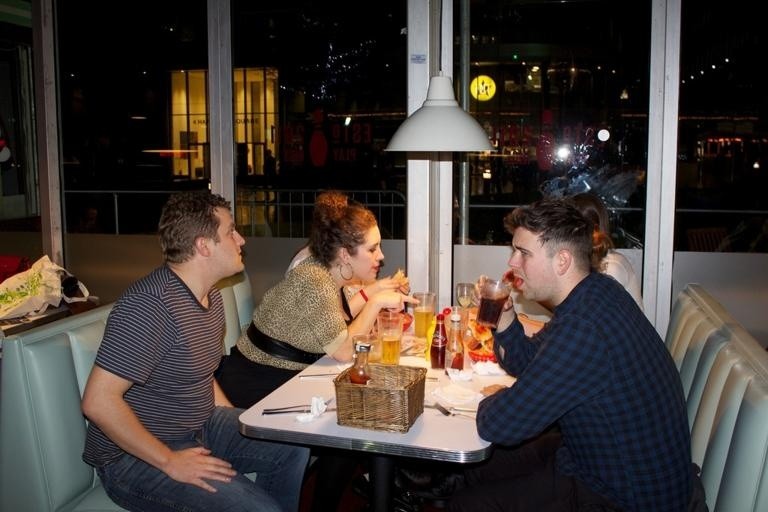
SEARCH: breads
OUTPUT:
[463,306,495,357]
[390,268,410,288]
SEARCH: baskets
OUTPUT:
[334,363,427,432]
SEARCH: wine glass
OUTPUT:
[457,283,476,308]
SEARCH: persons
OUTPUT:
[212,187,420,511]
[448,192,692,510]
[82,190,311,512]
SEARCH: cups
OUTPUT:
[377,311,405,365]
[414,293,436,338]
[476,278,513,328]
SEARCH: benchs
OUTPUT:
[664,282,768,512]
[0,268,370,512]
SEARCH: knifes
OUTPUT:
[423,405,479,413]
[262,407,335,415]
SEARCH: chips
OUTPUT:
[401,334,428,357]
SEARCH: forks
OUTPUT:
[264,397,335,412]
[433,402,477,420]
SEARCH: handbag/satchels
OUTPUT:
[1,255,90,320]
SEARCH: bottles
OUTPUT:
[426,308,464,368]
[351,343,371,384]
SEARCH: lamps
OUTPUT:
[384,0,499,152]
